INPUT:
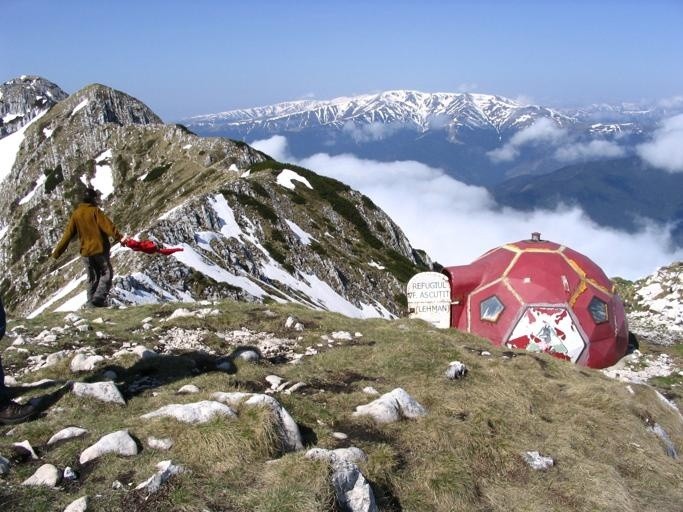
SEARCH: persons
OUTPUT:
[51,188,131,307]
[0,297,39,424]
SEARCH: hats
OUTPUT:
[84,189,97,197]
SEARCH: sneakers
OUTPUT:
[92,297,107,307]
